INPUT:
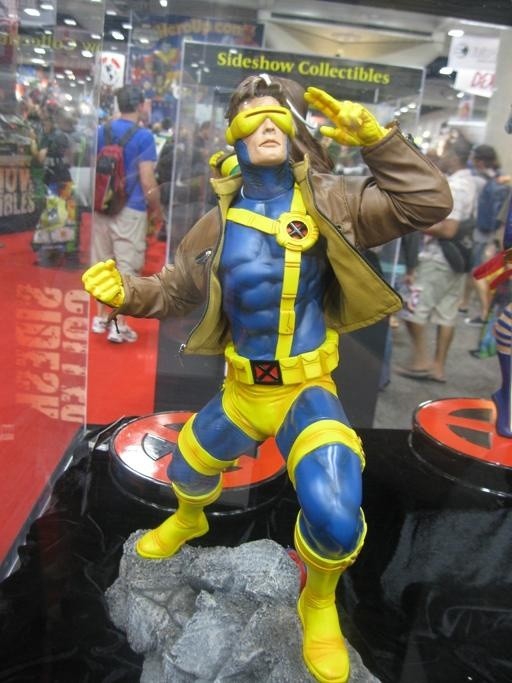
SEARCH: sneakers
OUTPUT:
[400,365,448,383]
[92,315,139,343]
[457,306,489,328]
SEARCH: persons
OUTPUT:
[81,72,454,683]
[309,84,512,383]
[1,84,238,343]
[471,248,512,439]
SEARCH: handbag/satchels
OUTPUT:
[439,215,477,274]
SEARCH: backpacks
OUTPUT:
[89,118,143,216]
[477,172,508,230]
